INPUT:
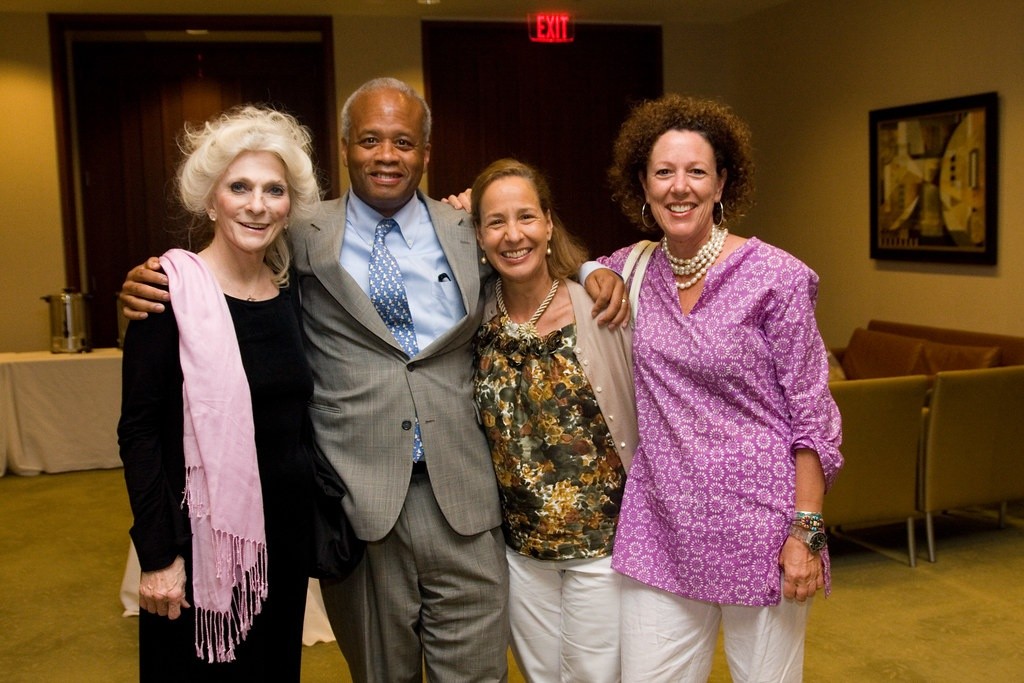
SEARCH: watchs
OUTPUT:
[789,525,828,553]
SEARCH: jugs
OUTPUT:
[40,288,95,354]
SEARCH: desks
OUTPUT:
[0,347,123,477]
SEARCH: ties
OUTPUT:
[368,219,424,464]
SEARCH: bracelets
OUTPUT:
[791,511,824,532]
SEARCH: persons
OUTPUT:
[470,158,639,683]
[120,77,632,683]
[117,104,318,683]
[441,89,846,683]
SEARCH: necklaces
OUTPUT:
[209,244,261,301]
[662,223,728,290]
[496,276,559,341]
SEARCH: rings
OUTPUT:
[621,298,629,303]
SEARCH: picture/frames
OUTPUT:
[870,91,1000,266]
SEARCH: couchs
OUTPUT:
[828,320,1023,567]
